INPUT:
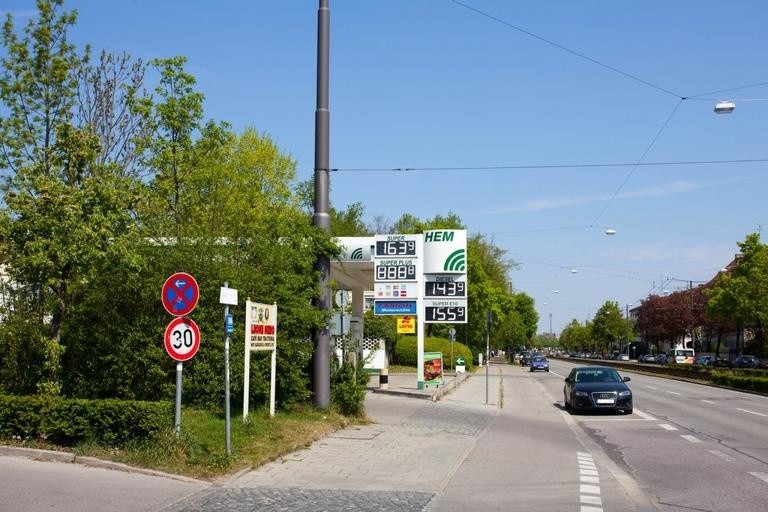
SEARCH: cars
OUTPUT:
[514,348,550,373]
[698,354,760,370]
[563,367,632,416]
[558,348,667,367]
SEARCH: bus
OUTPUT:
[665,349,695,363]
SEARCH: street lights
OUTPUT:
[625,303,634,356]
[719,265,741,273]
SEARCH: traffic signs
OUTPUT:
[164,318,200,361]
